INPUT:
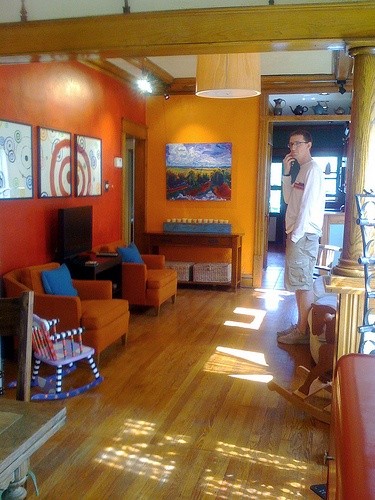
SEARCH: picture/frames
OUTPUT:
[74,134,102,197]
[1,120,33,199]
[37,127,73,198]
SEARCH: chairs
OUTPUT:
[9,313,104,401]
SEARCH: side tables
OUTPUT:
[75,256,122,299]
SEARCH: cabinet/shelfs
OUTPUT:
[324,354,375,500]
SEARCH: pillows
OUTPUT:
[40,265,77,297]
[117,243,143,263]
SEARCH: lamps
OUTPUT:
[195,55,261,99]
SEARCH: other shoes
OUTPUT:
[276,322,310,345]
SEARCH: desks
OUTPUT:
[142,233,243,293]
[0,398,67,500]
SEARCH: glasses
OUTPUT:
[288,141,307,149]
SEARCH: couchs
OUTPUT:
[122,254,177,317]
[2,263,130,368]
[308,275,338,364]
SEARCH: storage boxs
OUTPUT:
[165,261,194,281]
[193,263,231,284]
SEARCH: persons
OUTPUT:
[278,130,326,345]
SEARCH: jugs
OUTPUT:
[273,98,287,116]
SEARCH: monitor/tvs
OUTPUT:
[53,205,92,261]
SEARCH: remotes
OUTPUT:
[95,254,118,256]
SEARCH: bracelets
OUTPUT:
[284,174,291,176]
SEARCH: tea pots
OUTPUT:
[332,106,346,115]
[289,105,307,115]
[309,101,328,115]
